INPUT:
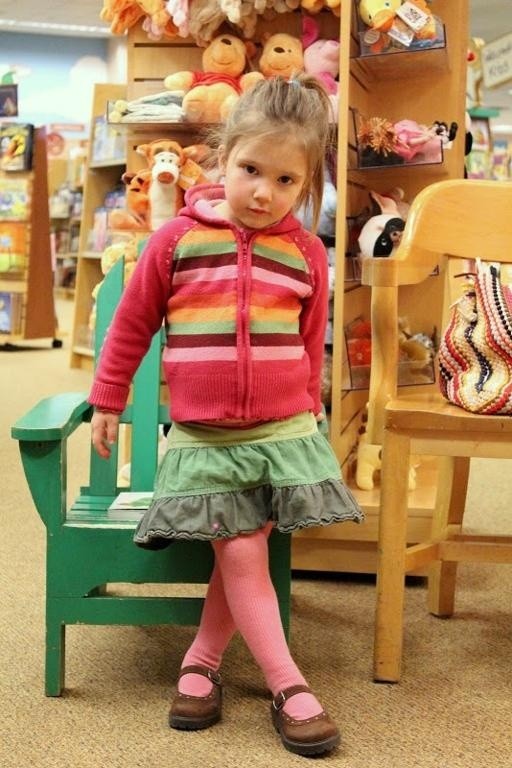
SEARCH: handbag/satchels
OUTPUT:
[438,260,511,415]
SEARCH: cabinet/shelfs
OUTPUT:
[0,123,58,351]
[69,3,466,583]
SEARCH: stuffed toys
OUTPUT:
[353,399,415,491]
[359,0,436,53]
[285,165,337,235]
[342,316,372,366]
[347,191,406,260]
[259,28,304,83]
[396,314,433,369]
[110,136,204,232]
[363,113,458,165]
[91,239,140,332]
[303,38,341,126]
[164,34,265,127]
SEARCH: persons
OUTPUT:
[89,74,361,758]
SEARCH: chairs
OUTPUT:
[9,244,312,700]
[360,179,511,683]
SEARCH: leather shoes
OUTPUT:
[168,664,225,731]
[267,683,340,756]
